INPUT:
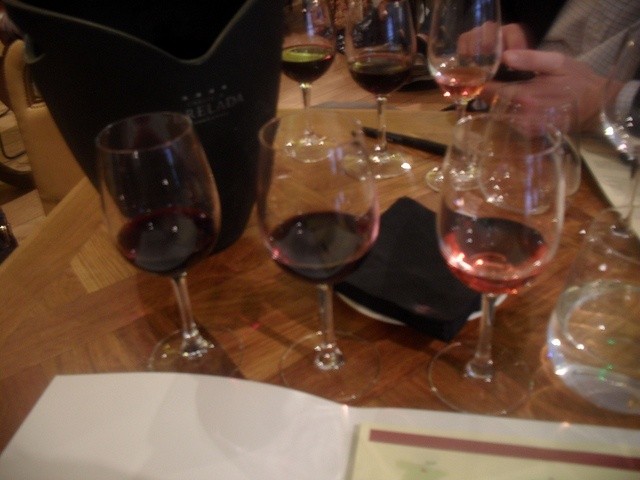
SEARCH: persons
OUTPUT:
[456,0,640,140]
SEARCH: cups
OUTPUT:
[476,81,582,219]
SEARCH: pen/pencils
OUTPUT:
[360,125,464,161]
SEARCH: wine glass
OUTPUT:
[421,0,506,193]
[95,110,247,379]
[338,0,420,179]
[576,28,640,253]
[425,112,571,420]
[530,207,640,429]
[253,106,387,401]
[276,1,335,163]
